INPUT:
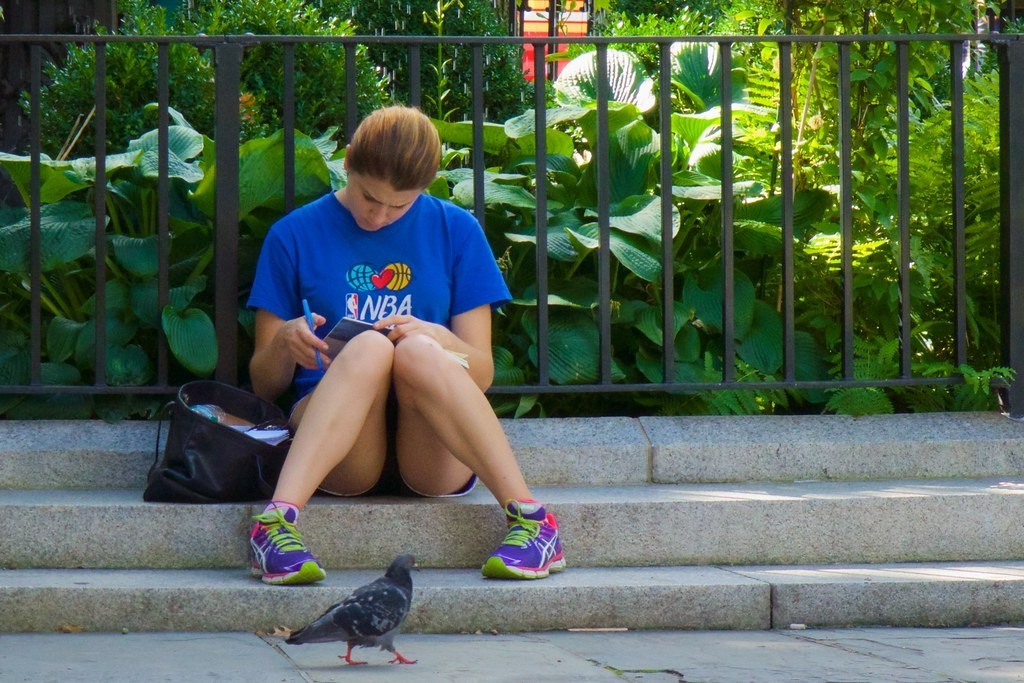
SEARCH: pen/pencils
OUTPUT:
[302,298,325,373]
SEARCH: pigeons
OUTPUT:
[286,553,421,667]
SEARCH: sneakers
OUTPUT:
[250,509,328,583]
[481,498,567,581]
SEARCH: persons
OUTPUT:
[245,106,565,587]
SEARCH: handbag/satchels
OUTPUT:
[143,380,298,504]
